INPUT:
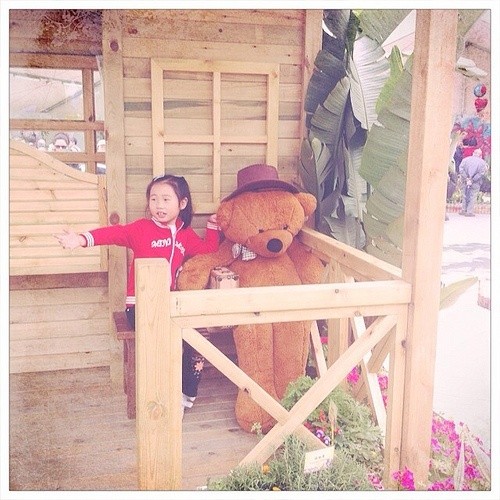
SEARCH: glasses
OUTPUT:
[54,145,66,148]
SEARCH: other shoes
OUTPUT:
[459,210,474,217]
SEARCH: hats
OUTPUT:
[221,164,298,204]
[53,133,69,142]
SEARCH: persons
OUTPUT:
[15,118,106,179]
[54,172,224,427]
[442,104,491,224]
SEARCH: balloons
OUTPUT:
[475,83,487,98]
[472,100,488,113]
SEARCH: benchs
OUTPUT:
[114,309,233,419]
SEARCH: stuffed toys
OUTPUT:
[178,162,330,437]
